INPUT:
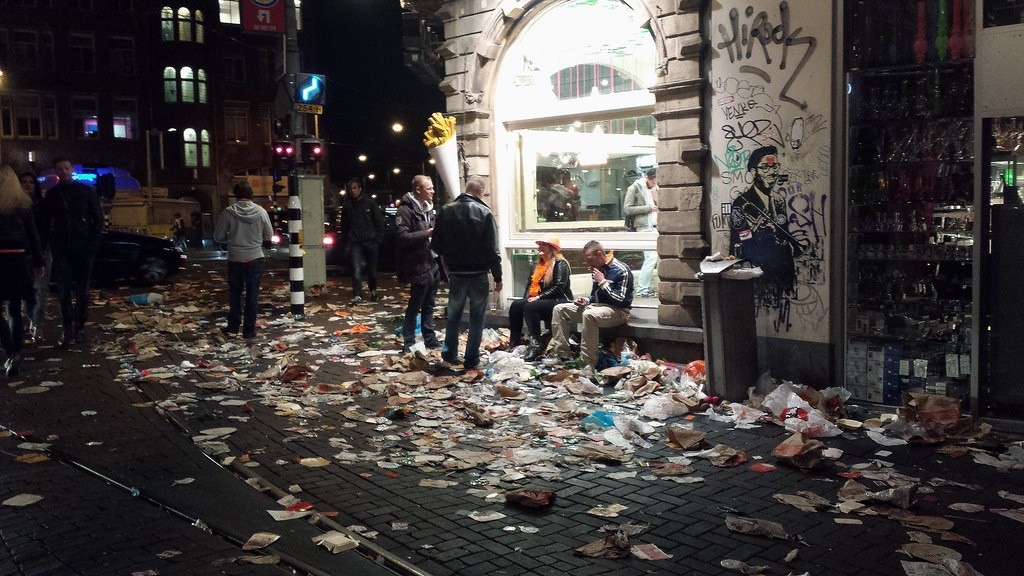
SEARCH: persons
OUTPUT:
[544,237,634,367]
[0,162,44,377]
[168,213,185,240]
[16,171,52,345]
[340,179,387,305]
[546,175,584,222]
[502,235,573,361]
[623,168,659,297]
[394,174,444,355]
[43,155,104,345]
[213,182,274,340]
[429,181,504,371]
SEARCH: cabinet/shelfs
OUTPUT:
[849,59,973,398]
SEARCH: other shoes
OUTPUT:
[61,323,72,343]
[41,314,53,320]
[21,325,35,345]
[75,322,85,343]
[441,351,455,364]
[542,354,571,366]
[220,327,237,337]
[425,341,443,350]
[3,353,21,377]
[403,343,414,355]
[250,331,257,338]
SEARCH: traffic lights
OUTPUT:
[301,139,323,159]
[292,72,327,114]
[272,140,294,158]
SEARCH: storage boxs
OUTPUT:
[843,342,900,404]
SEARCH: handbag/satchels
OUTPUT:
[262,230,271,250]
[624,185,638,228]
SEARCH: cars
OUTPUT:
[262,205,345,249]
[46,223,187,295]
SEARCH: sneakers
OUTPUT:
[370,290,379,301]
[349,296,363,305]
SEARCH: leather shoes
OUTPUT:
[505,341,520,352]
[523,345,542,361]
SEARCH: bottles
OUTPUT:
[857,274,936,296]
[848,303,971,346]
[541,356,561,366]
[852,166,973,203]
[856,206,974,233]
[845,403,869,414]
[481,368,494,376]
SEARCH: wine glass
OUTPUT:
[855,69,970,120]
[858,125,973,162]
[991,118,1024,152]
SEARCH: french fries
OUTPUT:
[424,112,457,148]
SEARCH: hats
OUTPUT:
[535,233,561,251]
[173,213,181,218]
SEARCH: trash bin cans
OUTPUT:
[698,259,758,403]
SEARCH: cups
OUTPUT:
[857,236,972,260]
